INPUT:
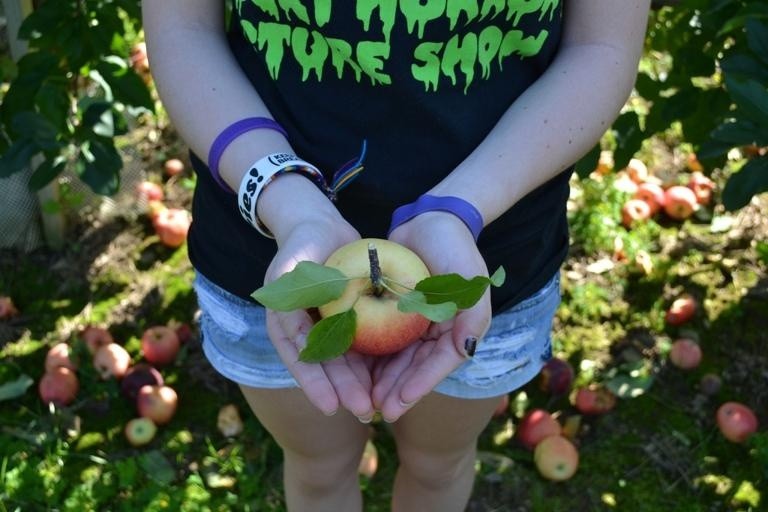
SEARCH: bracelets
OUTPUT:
[238,152,330,241]
[208,116,289,197]
[384,193,484,247]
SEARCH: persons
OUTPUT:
[138,1,654,510]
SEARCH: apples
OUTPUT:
[39,327,180,446]
[596,152,713,231]
[670,338,703,369]
[0,297,12,317]
[135,158,192,247]
[318,237,433,357]
[716,402,758,443]
[493,357,616,482]
[131,42,149,75]
